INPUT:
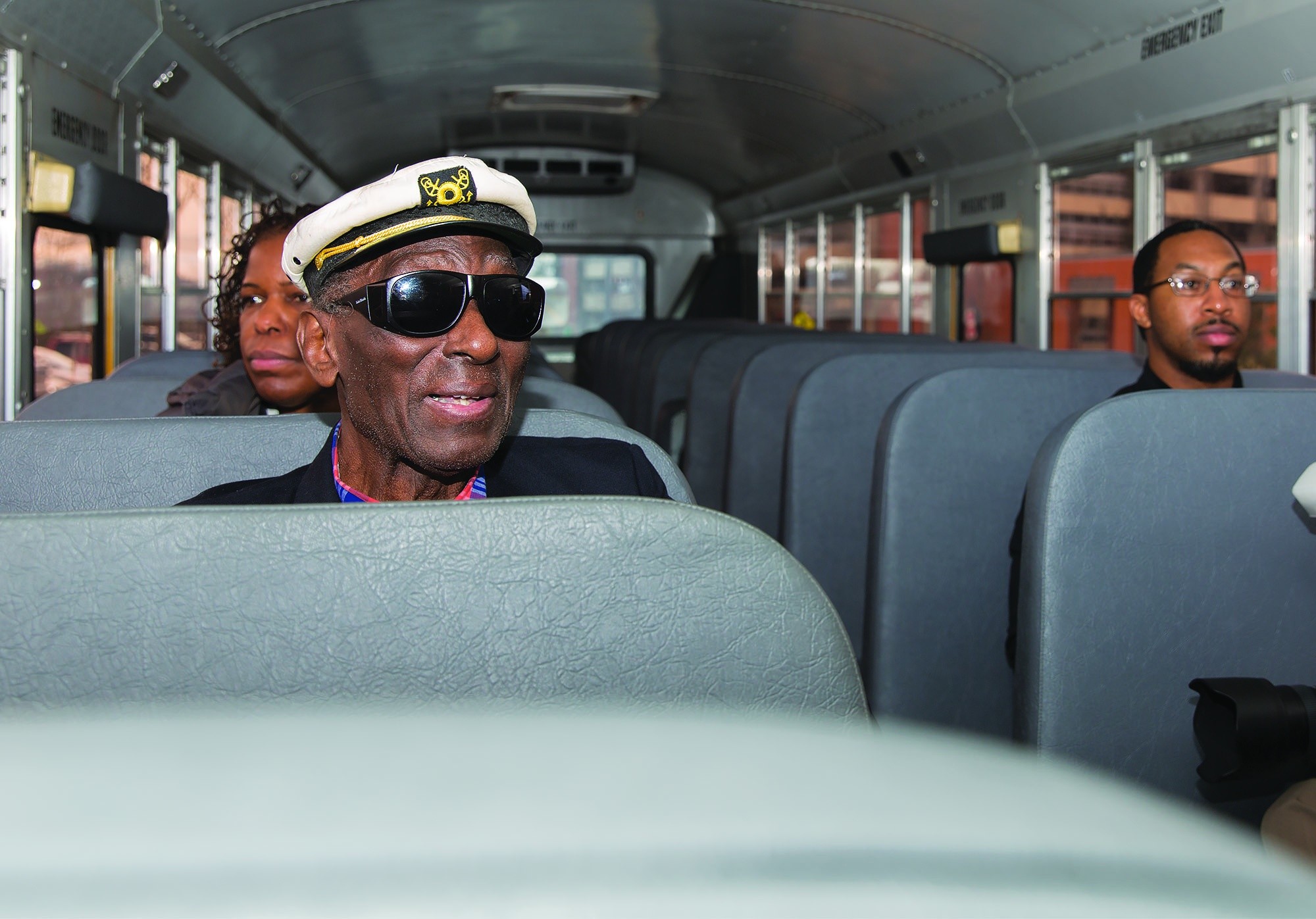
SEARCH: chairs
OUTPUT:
[0,316,1315,918]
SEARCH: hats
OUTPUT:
[281,158,543,300]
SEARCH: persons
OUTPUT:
[1005,220,1261,666]
[173,155,674,508]
[156,200,341,417]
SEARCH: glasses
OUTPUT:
[1142,273,1259,297]
[322,270,545,341]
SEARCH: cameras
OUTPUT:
[1188,678,1316,803]
